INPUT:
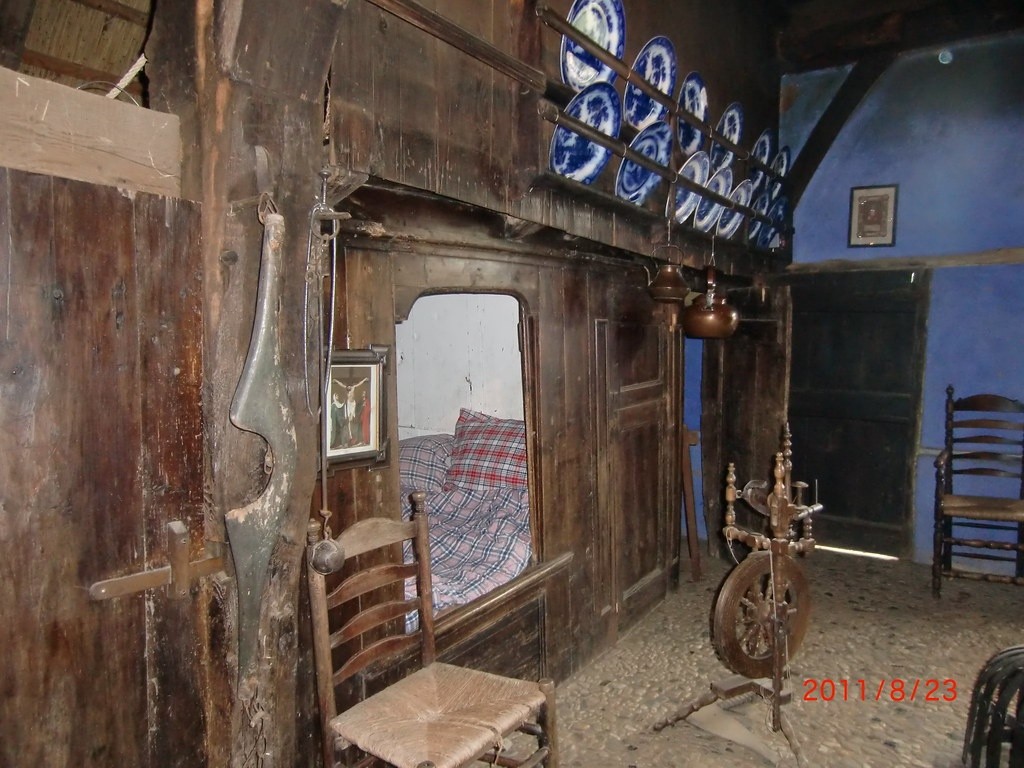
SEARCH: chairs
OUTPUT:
[304,490,557,768]
[931,384,1024,600]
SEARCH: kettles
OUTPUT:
[681,266,739,339]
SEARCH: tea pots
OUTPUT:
[643,246,688,304]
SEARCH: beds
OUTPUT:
[325,235,602,768]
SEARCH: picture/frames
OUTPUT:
[315,344,391,480]
[848,183,899,247]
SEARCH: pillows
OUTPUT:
[441,408,528,490]
[400,433,453,492]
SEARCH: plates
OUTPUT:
[561,0,625,93]
[693,166,733,233]
[678,71,709,159]
[548,81,622,185]
[624,36,677,131]
[731,193,770,241]
[664,151,709,225]
[765,145,791,202]
[745,128,770,191]
[757,200,787,250]
[710,102,743,176]
[716,179,753,239]
[615,122,673,206]
